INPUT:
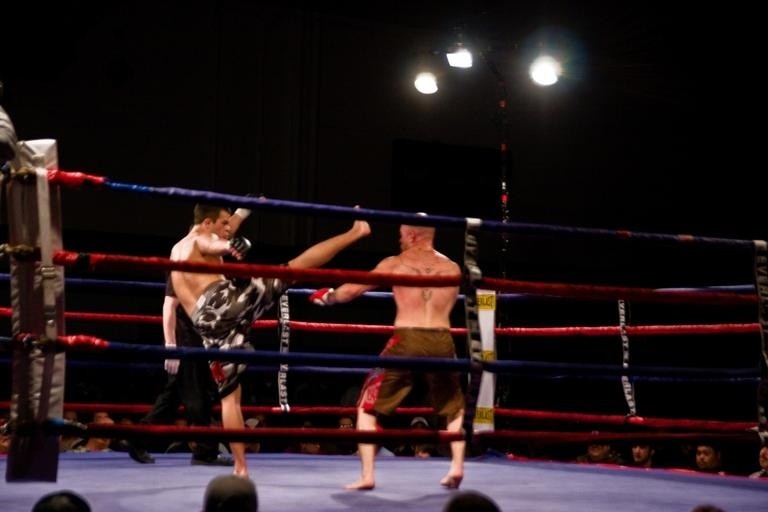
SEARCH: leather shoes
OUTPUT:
[120,439,155,464]
[191,447,234,466]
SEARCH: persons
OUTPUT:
[1,403,768,482]
[443,490,501,512]
[309,212,467,491]
[203,476,259,512]
[127,271,235,466]
[32,489,91,511]
[170,192,372,479]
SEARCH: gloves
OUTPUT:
[308,287,334,307]
[228,236,252,260]
